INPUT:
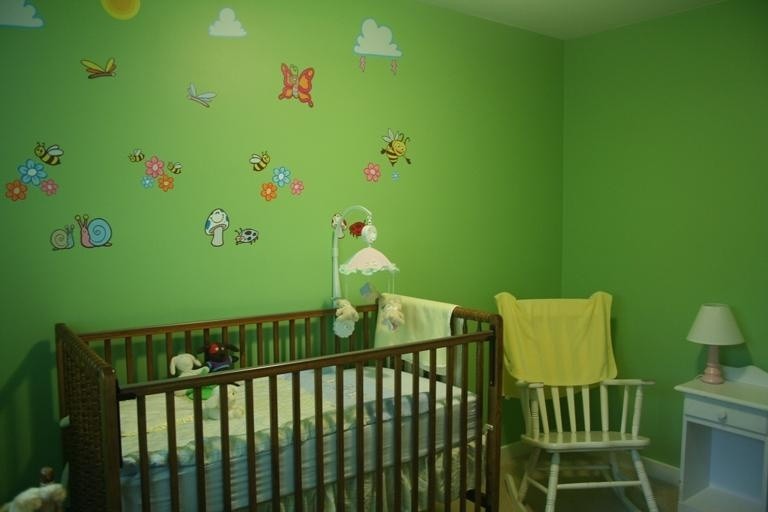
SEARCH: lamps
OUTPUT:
[687,303,744,384]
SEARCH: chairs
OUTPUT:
[519,377,661,512]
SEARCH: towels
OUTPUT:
[374,292,465,386]
[495,290,618,402]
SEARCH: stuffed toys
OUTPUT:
[201,381,245,422]
[379,295,406,333]
[169,353,211,397]
[199,342,241,387]
[335,299,359,322]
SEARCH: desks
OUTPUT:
[675,364,768,512]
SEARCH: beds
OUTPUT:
[54,296,501,512]
